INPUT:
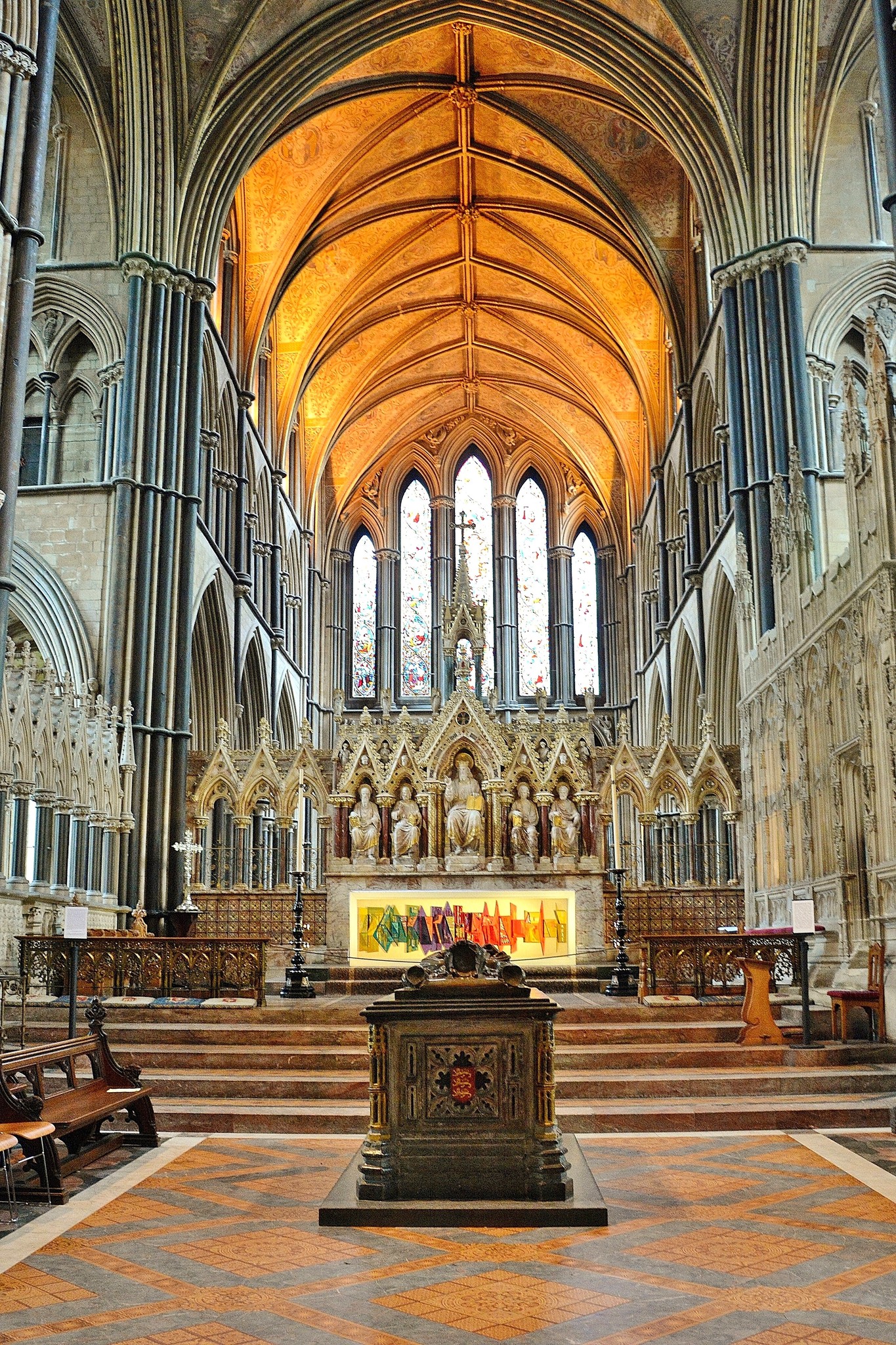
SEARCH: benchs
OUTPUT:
[1,995,161,1205]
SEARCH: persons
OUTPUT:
[442,761,485,854]
[361,755,369,765]
[558,753,567,765]
[380,689,391,718]
[577,741,590,768]
[584,685,596,712]
[535,685,547,713]
[644,776,654,789]
[333,688,344,718]
[339,743,351,766]
[348,787,382,863]
[548,782,581,858]
[537,741,549,765]
[380,743,390,765]
[508,782,540,862]
[401,755,409,767]
[520,754,527,766]
[686,776,695,787]
[390,787,422,862]
[487,685,499,715]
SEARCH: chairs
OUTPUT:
[0,1121,55,1213]
[827,941,884,1044]
[0,1131,19,1223]
